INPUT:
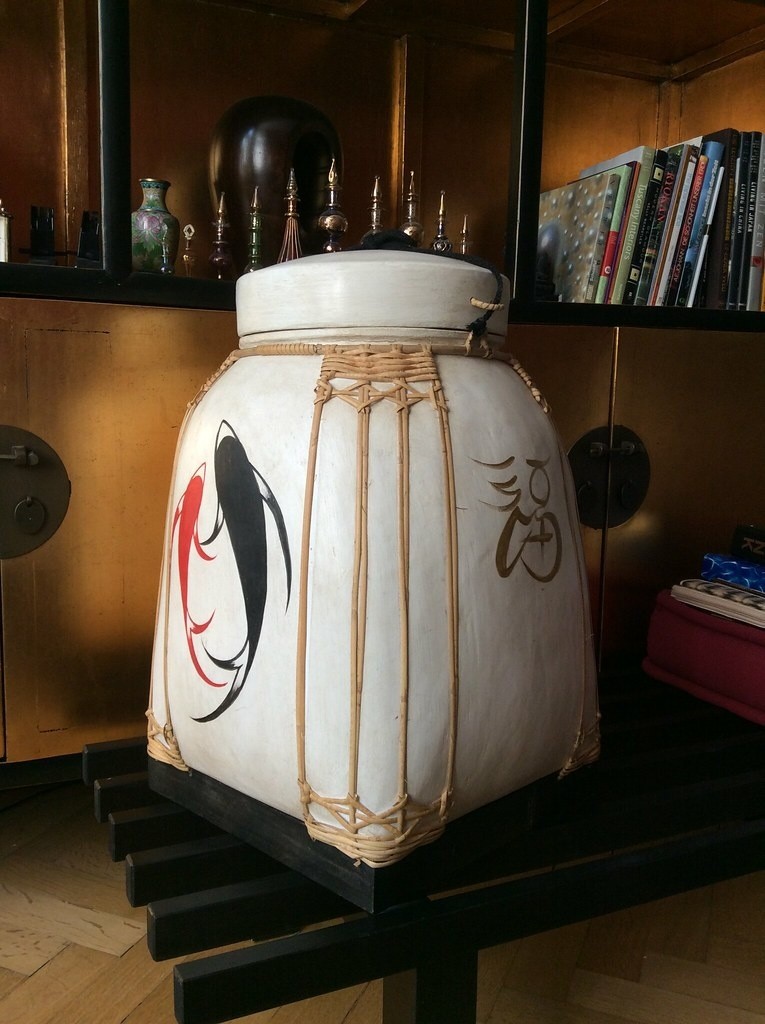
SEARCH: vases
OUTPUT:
[132,179,180,274]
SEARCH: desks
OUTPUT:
[82,665,765,1024]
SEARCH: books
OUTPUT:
[669,525,765,632]
[538,128,765,312]
